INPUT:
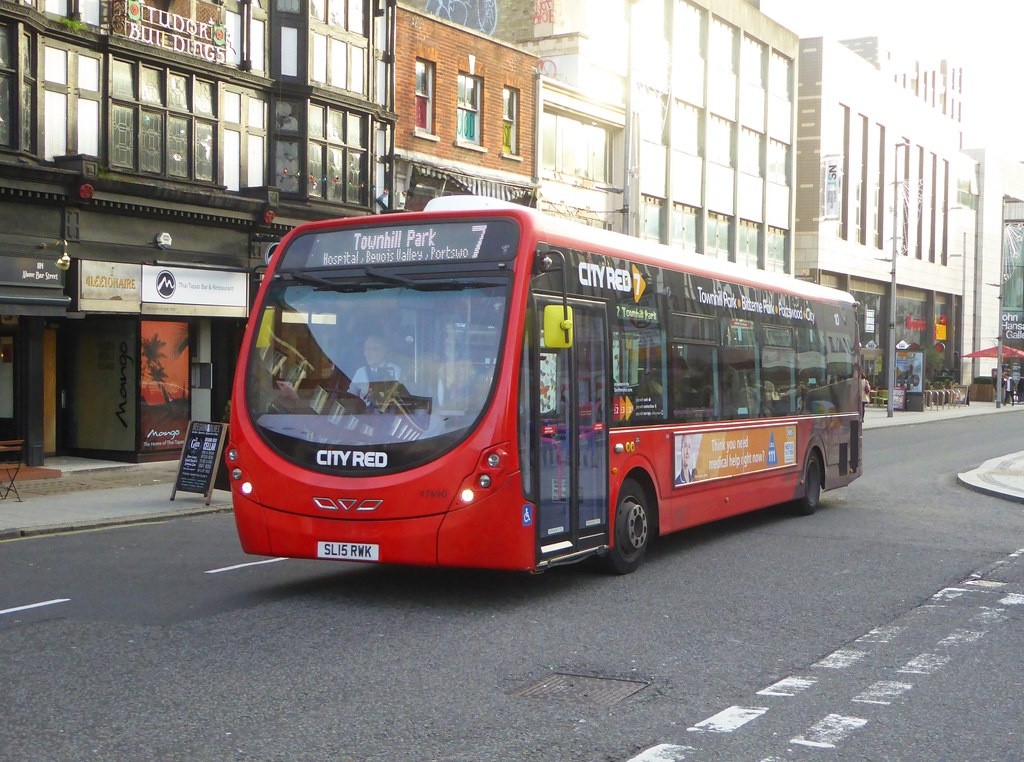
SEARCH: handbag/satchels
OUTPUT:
[864,380,870,395]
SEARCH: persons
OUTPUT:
[992,367,1024,406]
[860,371,870,422]
[637,380,684,411]
[346,334,403,406]
[719,350,740,393]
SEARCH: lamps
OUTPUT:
[41,239,70,270]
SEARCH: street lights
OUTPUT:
[887,140,907,418]
[996,194,1024,408]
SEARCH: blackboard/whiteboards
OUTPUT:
[174,421,229,494]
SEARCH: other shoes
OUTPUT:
[862,418,864,422]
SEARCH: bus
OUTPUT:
[224,194,863,576]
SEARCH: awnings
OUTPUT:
[413,162,542,200]
[962,344,1024,358]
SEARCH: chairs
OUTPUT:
[0,440,24,502]
[871,390,889,409]
